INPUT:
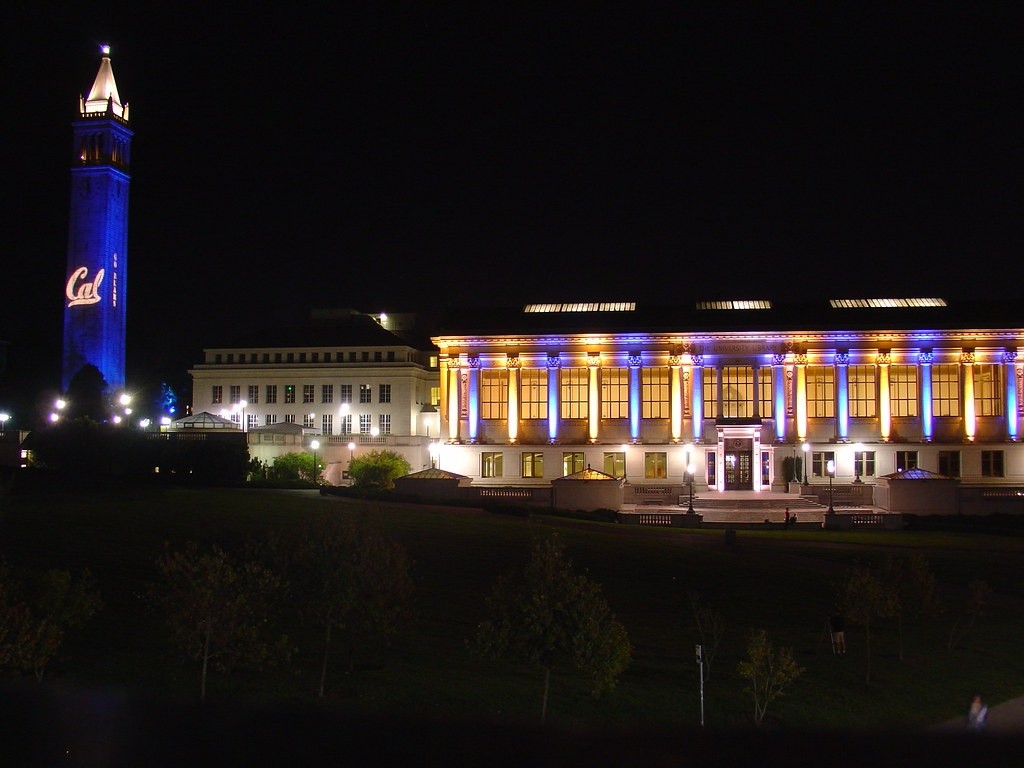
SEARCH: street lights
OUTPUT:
[429,443,436,468]
[687,464,696,515]
[827,465,835,512]
[347,442,355,486]
[437,443,442,470]
[311,439,319,488]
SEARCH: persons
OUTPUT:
[832,608,847,655]
[784,507,797,531]
[968,694,990,734]
